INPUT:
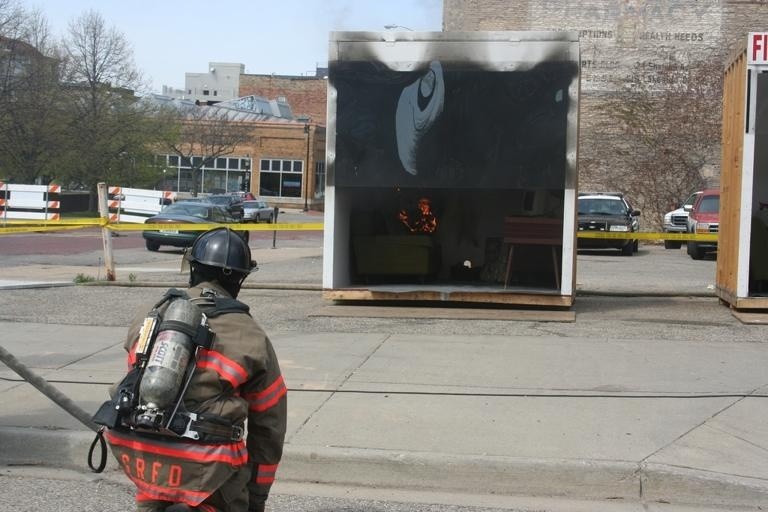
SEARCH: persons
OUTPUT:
[90,227,291,512]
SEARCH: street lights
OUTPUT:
[244,160,250,192]
[385,24,413,31]
[300,125,313,211]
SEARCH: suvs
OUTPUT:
[663,192,703,250]
[684,190,719,261]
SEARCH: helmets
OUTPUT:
[185,224,259,275]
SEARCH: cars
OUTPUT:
[576,192,642,257]
[142,191,275,256]
